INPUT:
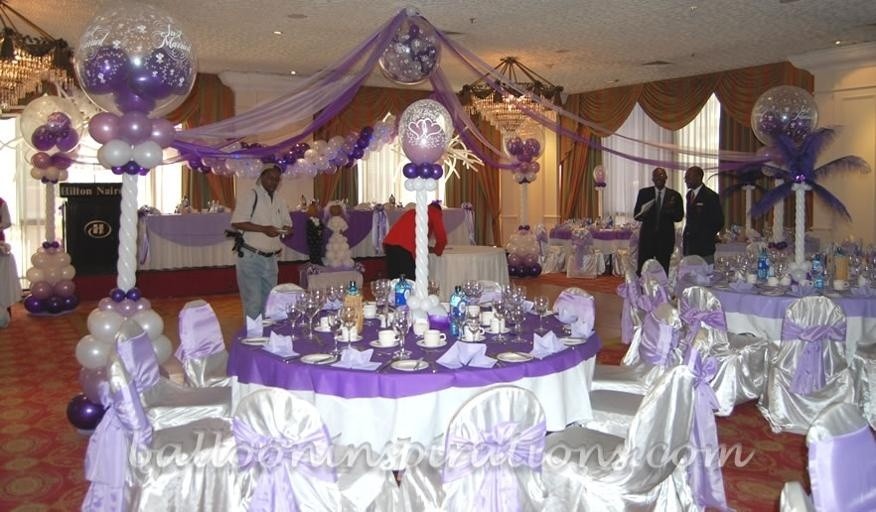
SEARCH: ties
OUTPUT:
[690,192,694,204]
[655,192,661,230]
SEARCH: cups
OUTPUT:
[207,199,218,214]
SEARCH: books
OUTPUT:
[634,199,653,220]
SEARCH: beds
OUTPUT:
[136,206,470,274]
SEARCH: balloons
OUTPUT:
[396,99,455,192]
[176,114,399,180]
[70,1,199,177]
[504,224,541,278]
[19,95,83,184]
[504,123,547,185]
[402,296,451,330]
[66,288,172,432]
[749,85,818,180]
[23,240,79,315]
[593,165,607,191]
[777,258,814,287]
[373,5,442,87]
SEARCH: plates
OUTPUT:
[275,229,289,234]
[557,337,586,348]
[242,338,272,347]
[391,361,429,372]
[300,353,335,366]
[497,352,534,364]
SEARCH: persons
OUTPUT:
[632,167,684,279]
[323,199,356,269]
[0,196,23,329]
[380,202,448,280]
[682,165,724,263]
[229,162,293,324]
[305,203,328,269]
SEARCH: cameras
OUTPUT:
[224,229,244,257]
[276,230,288,239]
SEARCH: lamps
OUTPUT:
[0,0,74,117]
[458,57,564,142]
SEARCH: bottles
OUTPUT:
[584,217,612,228]
[181,196,191,215]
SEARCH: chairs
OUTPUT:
[83,226,876,512]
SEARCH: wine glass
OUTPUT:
[720,240,875,290]
[282,276,550,357]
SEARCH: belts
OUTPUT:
[242,243,282,257]
